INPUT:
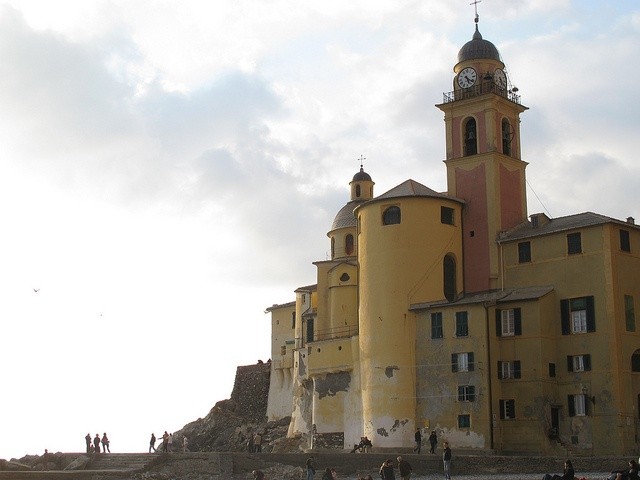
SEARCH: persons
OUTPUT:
[429,431,437,455]
[149,434,159,453]
[85,434,91,452]
[166,433,173,452]
[397,456,412,480]
[182,435,190,452]
[321,467,337,480]
[159,431,169,452]
[443,442,451,479]
[351,436,372,454]
[543,460,574,480]
[44,449,49,470]
[415,427,422,455]
[246,433,254,452]
[252,470,264,480]
[608,460,638,480]
[305,458,314,480]
[101,433,110,453]
[310,456,315,474]
[255,433,262,453]
[379,459,395,479]
[94,434,100,453]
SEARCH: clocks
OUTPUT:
[456,67,477,89]
[494,68,507,89]
